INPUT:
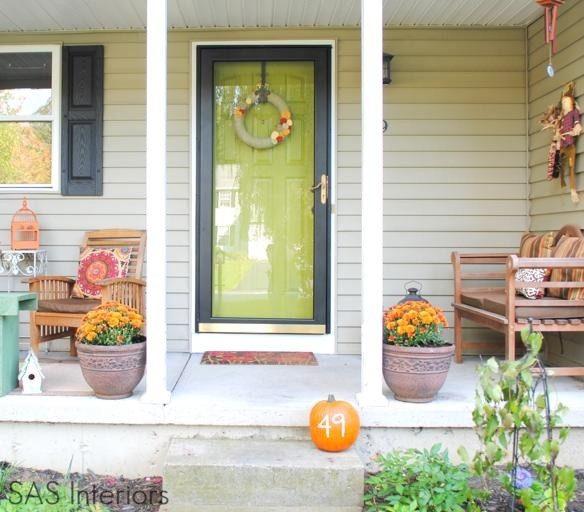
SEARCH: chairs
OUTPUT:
[20,229,147,360]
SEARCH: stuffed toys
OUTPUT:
[550,93,583,205]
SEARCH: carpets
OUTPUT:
[200,351,319,366]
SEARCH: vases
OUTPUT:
[75,334,147,399]
[383,342,455,403]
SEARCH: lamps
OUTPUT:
[383,52,395,84]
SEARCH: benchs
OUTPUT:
[451,223,584,377]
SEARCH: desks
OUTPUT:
[0,291,39,399]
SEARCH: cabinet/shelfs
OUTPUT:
[0,250,48,353]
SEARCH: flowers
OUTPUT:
[233,88,292,149]
[76,300,144,345]
[383,300,448,348]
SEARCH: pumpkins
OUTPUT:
[309,393,359,452]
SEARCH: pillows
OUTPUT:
[515,267,552,299]
[546,235,584,300]
[519,230,553,258]
[70,243,133,300]
